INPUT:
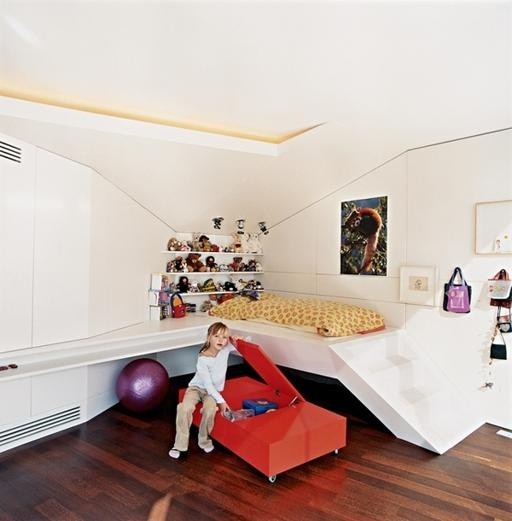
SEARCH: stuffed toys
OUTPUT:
[159,232,264,309]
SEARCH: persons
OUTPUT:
[168,321,253,458]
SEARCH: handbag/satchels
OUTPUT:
[443,267,471,313]
[487,269,512,308]
[170,292,186,318]
[497,305,512,333]
[491,325,506,359]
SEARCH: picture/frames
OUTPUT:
[475,199,512,255]
[400,264,436,306]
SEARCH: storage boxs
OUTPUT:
[177,336,347,482]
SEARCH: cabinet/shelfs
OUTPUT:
[147,232,264,322]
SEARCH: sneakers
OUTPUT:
[204,444,215,454]
[168,449,180,459]
